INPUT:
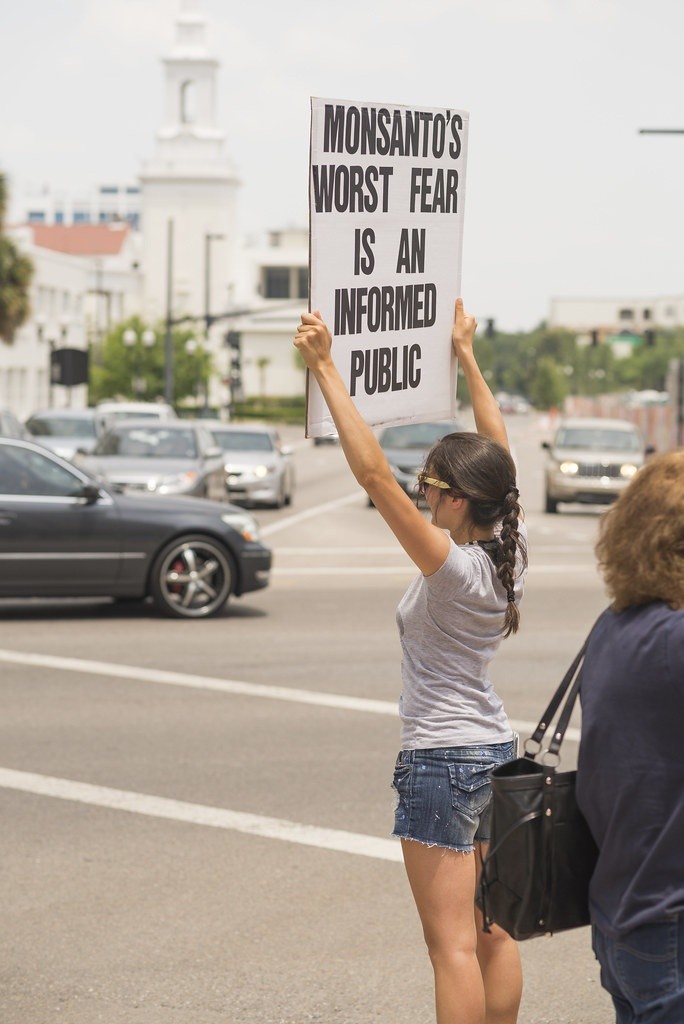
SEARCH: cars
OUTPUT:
[0,407,64,458]
[208,424,296,509]
[23,410,100,452]
[94,403,177,435]
[2,437,275,618]
[536,418,656,516]
[70,422,223,500]
[367,420,464,512]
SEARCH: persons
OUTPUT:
[295,299,522,1024]
[574,451,683,1024]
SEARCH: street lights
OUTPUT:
[198,230,227,418]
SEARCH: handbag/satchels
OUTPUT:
[475,603,600,942]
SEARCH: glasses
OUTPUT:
[418,472,452,496]
